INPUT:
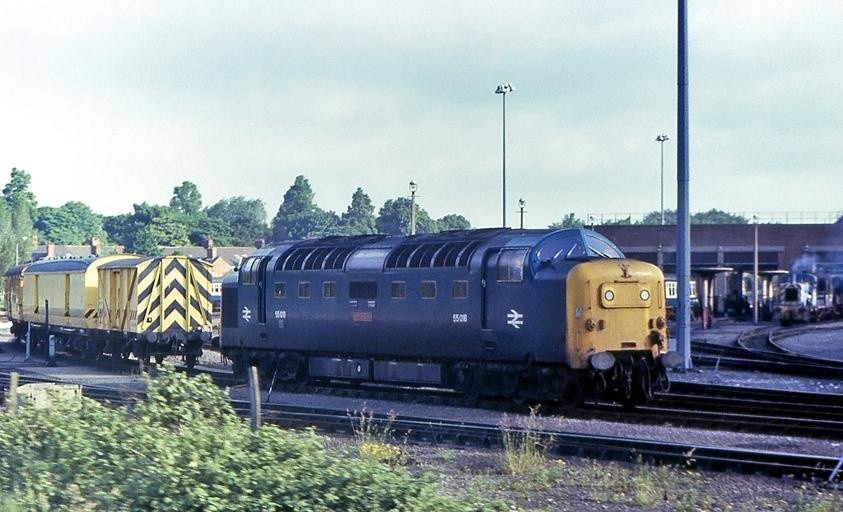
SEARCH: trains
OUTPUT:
[780,271,843,324]
[0,226,673,416]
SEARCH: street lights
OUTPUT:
[494,81,515,228]
[408,181,418,236]
[518,197,526,229]
[656,134,670,225]
